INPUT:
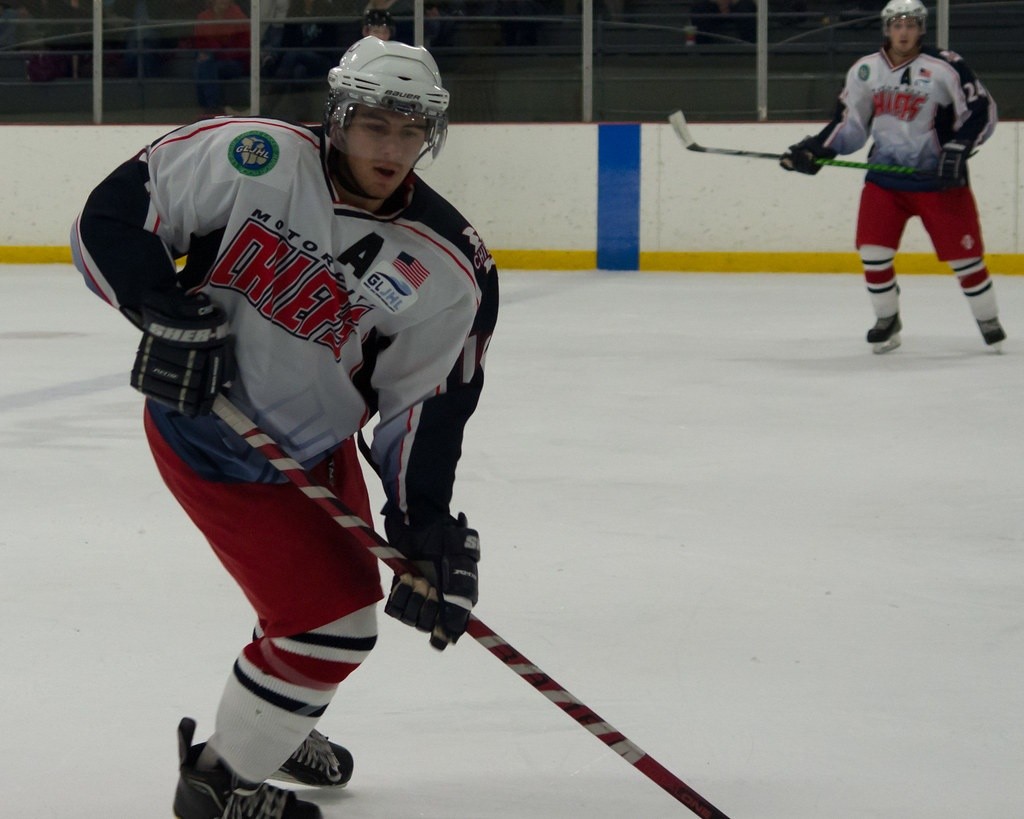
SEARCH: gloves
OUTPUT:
[381,513,480,652]
[931,138,979,188]
[780,136,838,176]
[132,293,238,421]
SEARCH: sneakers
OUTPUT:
[267,727,354,790]
[866,313,902,355]
[976,317,1007,355]
[173,717,324,819]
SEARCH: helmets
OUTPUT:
[881,0,928,37]
[326,35,450,143]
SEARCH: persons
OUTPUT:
[781,1,1009,354]
[189,3,253,109]
[69,37,499,819]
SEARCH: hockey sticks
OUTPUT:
[212,388,727,819]
[669,109,939,177]
